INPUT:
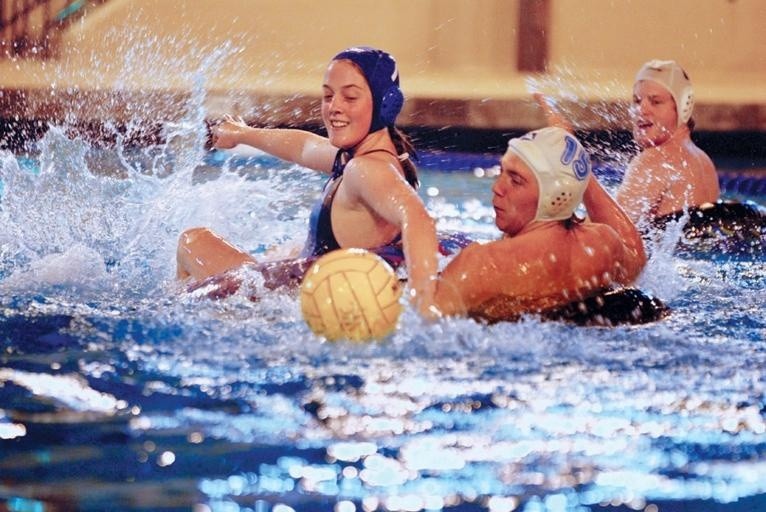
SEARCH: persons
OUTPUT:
[419,93,644,323]
[617,57,718,223]
[176,46,438,319]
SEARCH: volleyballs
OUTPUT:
[300,249,400,339]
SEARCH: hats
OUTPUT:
[634,58,695,130]
[330,45,404,135]
[508,125,592,223]
